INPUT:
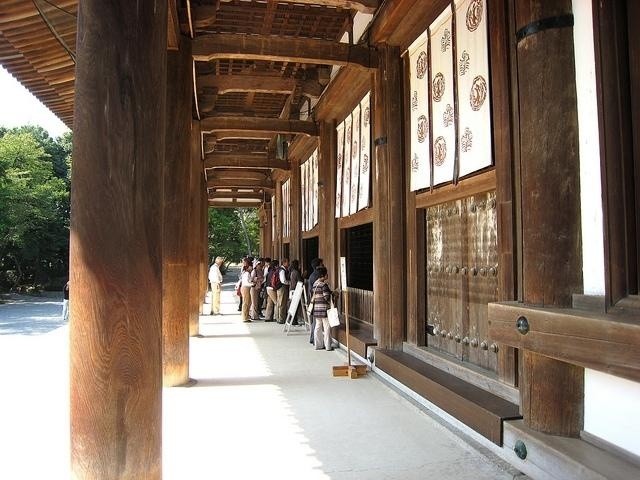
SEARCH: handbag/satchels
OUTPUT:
[307,296,314,316]
[259,282,271,299]
[326,308,341,328]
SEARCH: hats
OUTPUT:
[253,260,263,269]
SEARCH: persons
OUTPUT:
[236,256,339,353]
[62,279,69,321]
[208,256,225,317]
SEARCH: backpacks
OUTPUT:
[235,279,242,297]
